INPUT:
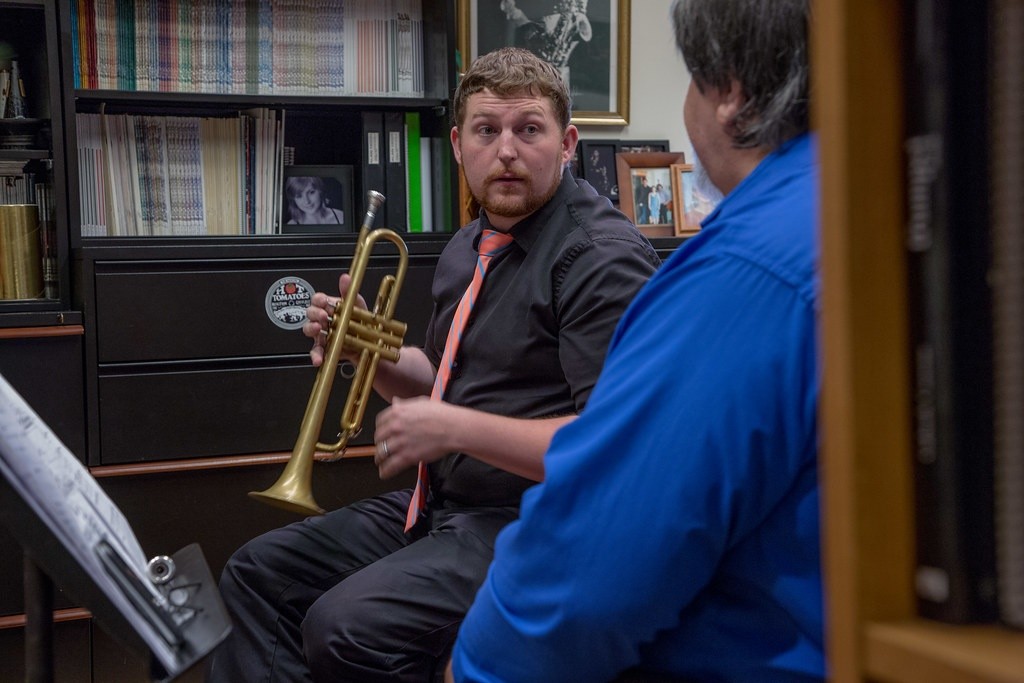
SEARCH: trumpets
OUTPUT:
[248,189,409,513]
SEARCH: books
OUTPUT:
[0,0,459,300]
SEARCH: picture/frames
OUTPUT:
[575,140,721,238]
[456,0,632,126]
[276,164,355,233]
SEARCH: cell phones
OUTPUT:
[95,540,183,647]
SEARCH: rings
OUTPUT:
[382,440,389,457]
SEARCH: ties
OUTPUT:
[404,227,514,535]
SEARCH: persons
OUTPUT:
[443,0,828,683]
[285,176,344,224]
[212,44,662,683]
[568,146,718,226]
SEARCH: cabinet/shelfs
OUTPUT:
[0,0,459,472]
